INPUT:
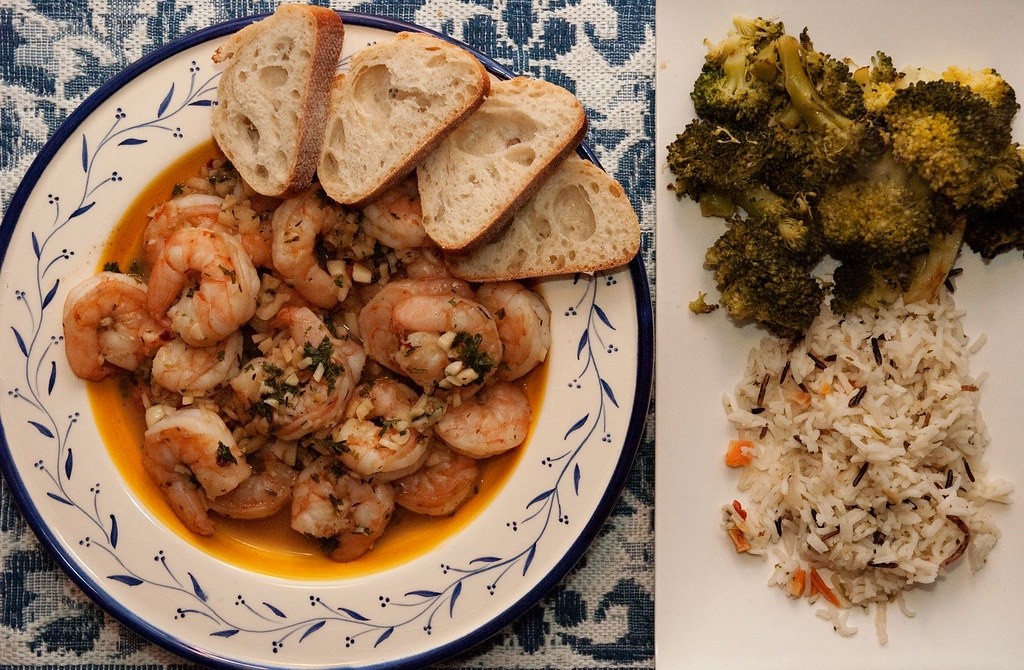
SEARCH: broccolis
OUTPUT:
[667,12,1024,339]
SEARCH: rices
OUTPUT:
[718,290,1018,647]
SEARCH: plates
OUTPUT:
[0,8,655,670]
[645,0,1024,670]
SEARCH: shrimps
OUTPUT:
[61,187,551,564]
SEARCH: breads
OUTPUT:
[211,0,642,281]
